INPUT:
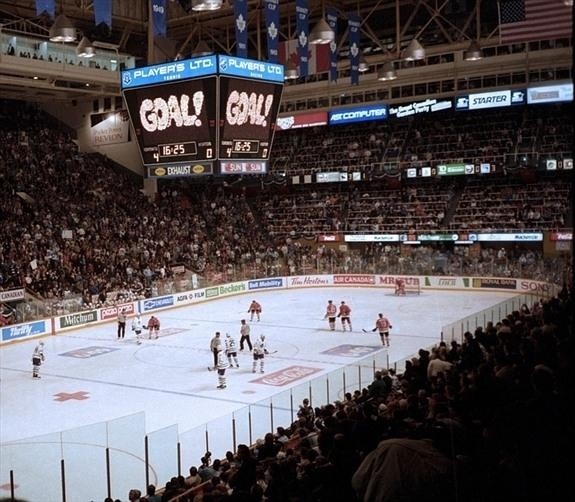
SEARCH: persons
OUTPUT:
[131,312,147,344]
[253,334,269,374]
[224,332,240,368]
[338,301,354,333]
[116,308,126,338]
[392,327,574,502]
[325,300,337,332]
[211,331,222,367]
[104,369,392,501]
[240,319,253,352]
[148,316,160,339]
[373,312,393,345]
[0,42,575,327]
[214,345,228,388]
[31,341,46,377]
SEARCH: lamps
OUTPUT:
[462,41,480,61]
[77,35,96,58]
[404,39,425,61]
[377,61,397,80]
[190,0,222,11]
[284,60,299,80]
[359,58,369,72]
[309,18,334,44]
[192,40,212,57]
[51,14,78,41]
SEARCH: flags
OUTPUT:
[497,1,573,46]
